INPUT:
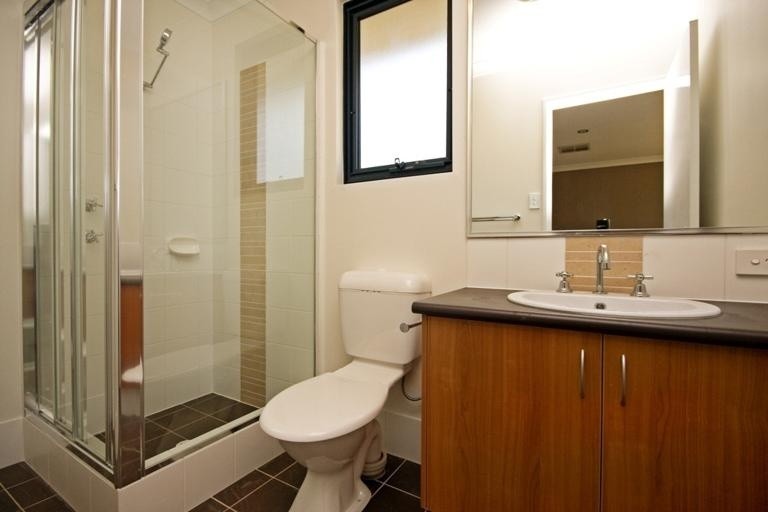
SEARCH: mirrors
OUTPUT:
[465,1,767,238]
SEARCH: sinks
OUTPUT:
[507,291,720,319]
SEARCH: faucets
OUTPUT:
[595,244,610,292]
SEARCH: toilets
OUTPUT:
[263,269,433,512]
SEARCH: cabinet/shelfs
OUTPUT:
[420,312,768,511]
